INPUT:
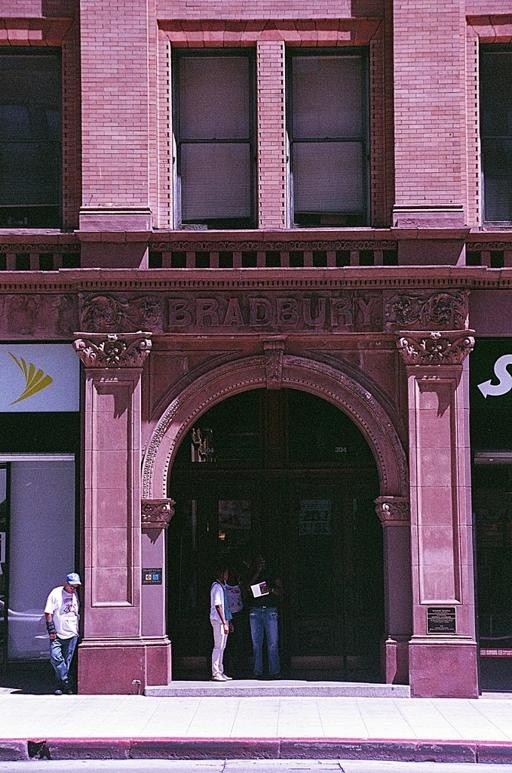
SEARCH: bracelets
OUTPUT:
[46,622,56,633]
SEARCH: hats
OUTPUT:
[66,572,82,589]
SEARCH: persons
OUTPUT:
[209,552,284,681]
[44,573,82,696]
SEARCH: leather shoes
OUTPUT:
[221,673,233,680]
[211,675,226,681]
[248,674,264,679]
[272,673,284,679]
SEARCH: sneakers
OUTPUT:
[54,686,63,695]
[63,686,75,694]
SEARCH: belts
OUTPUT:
[260,604,268,608]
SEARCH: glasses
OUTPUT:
[68,585,80,591]
